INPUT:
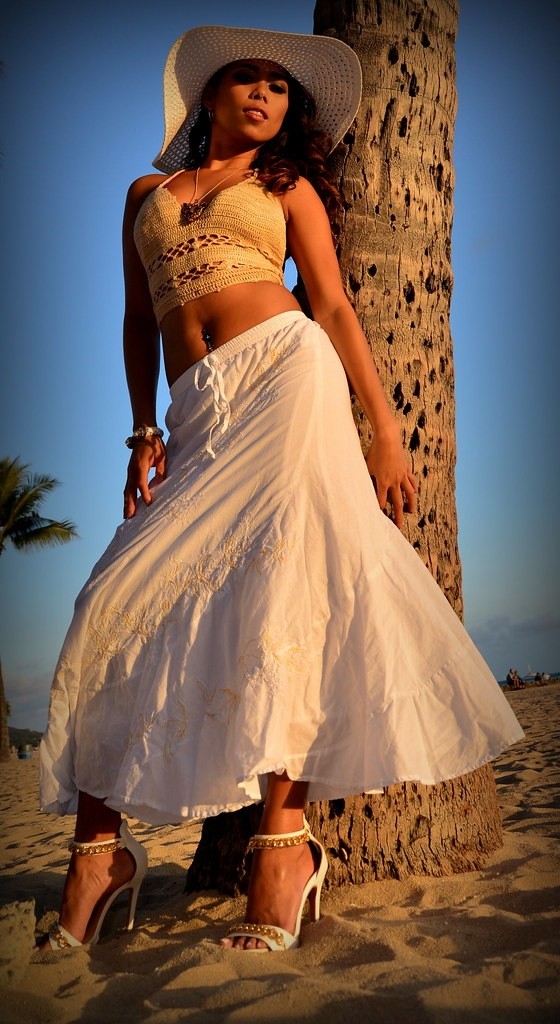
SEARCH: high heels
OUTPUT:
[216,813,329,954]
[36,818,149,953]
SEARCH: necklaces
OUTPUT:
[181,165,244,222]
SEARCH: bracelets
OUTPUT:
[125,426,163,449]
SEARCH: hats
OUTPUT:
[152,24,363,176]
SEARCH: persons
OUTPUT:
[534,672,547,686]
[507,668,525,691]
[40,25,527,953]
